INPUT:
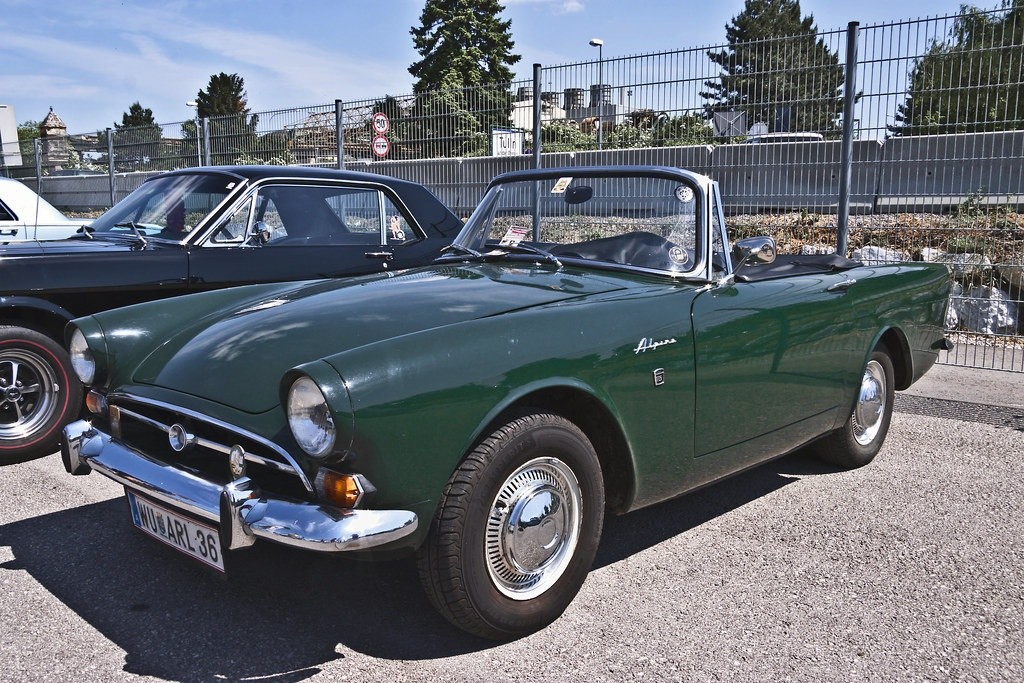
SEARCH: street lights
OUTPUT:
[588,37,604,150]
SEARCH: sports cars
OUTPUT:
[58,164,956,640]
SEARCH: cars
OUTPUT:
[2,174,161,241]
[0,163,465,466]
